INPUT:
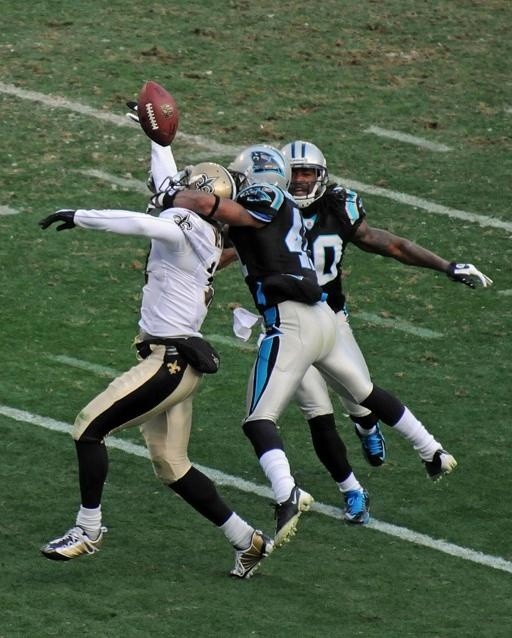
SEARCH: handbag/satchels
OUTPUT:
[174,335,221,374]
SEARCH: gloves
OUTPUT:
[446,261,494,289]
[39,208,76,231]
[145,188,179,214]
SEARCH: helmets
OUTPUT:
[227,145,291,192]
[159,162,236,226]
[280,140,329,208]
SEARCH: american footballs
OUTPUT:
[138,81,178,146]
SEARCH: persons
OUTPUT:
[212,140,494,527]
[37,100,275,581]
[145,142,458,549]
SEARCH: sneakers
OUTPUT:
[422,450,457,482]
[230,528,276,579]
[39,523,108,562]
[354,421,386,467]
[340,482,370,525]
[273,484,313,547]
